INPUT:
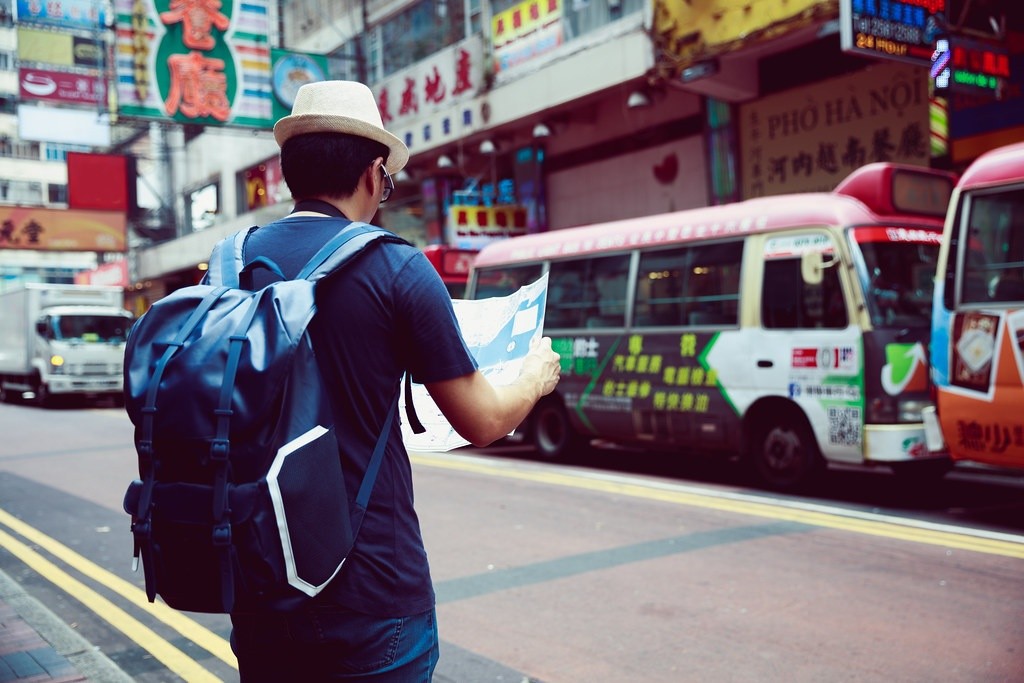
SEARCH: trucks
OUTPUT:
[1,282,136,410]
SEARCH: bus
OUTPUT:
[417,245,519,300]
[926,142,1023,475]
[460,162,960,497]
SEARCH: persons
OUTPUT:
[198,80,562,683]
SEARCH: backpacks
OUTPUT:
[121,221,411,614]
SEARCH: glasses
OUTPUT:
[371,159,394,202]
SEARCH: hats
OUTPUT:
[272,79,409,176]
